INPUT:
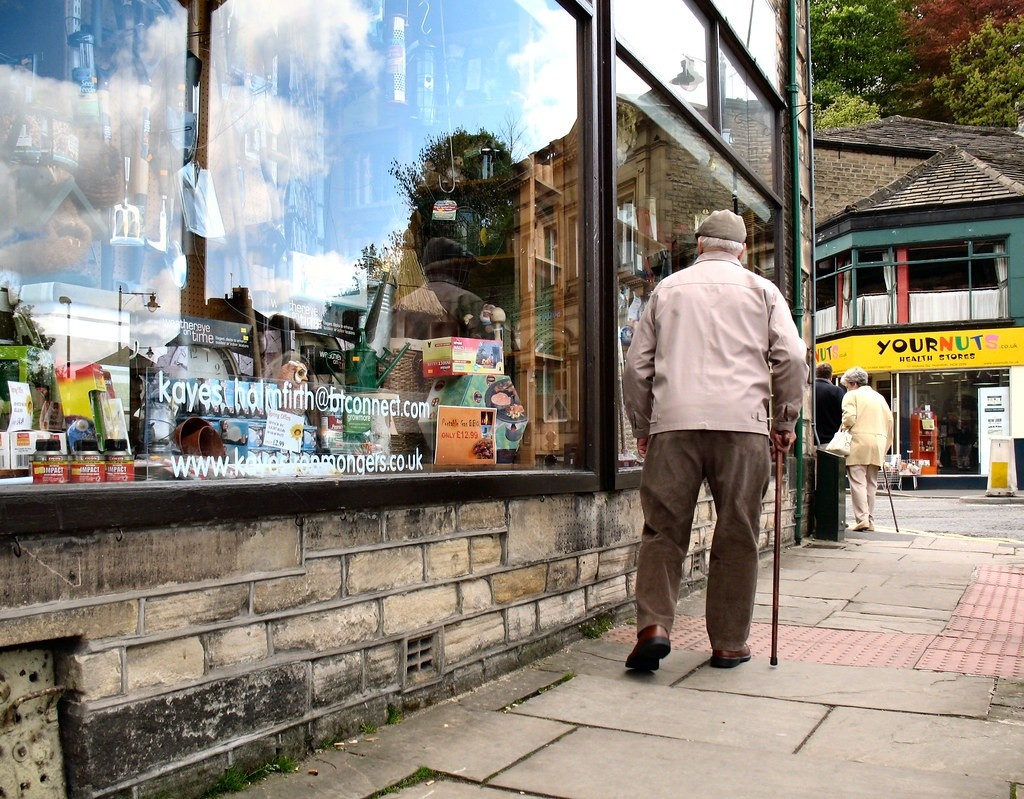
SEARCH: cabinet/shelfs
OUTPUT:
[1,343,116,457]
[565,209,667,468]
[416,124,566,467]
[910,413,937,475]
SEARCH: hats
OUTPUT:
[694,209,747,243]
[421,237,478,273]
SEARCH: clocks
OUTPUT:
[157,347,241,380]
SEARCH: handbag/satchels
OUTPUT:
[826,423,852,455]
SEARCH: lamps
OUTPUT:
[616,93,637,168]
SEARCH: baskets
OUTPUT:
[378,337,433,463]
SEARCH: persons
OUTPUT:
[812,362,894,533]
[622,210,810,672]
[389,237,488,339]
[950,382,977,468]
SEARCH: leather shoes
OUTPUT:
[711,643,751,668]
[624,625,671,670]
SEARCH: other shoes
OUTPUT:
[853,521,869,531]
[867,524,874,531]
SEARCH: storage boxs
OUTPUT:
[300,345,343,374]
[218,419,317,465]
[165,315,253,349]
[421,337,504,379]
[417,375,528,463]
